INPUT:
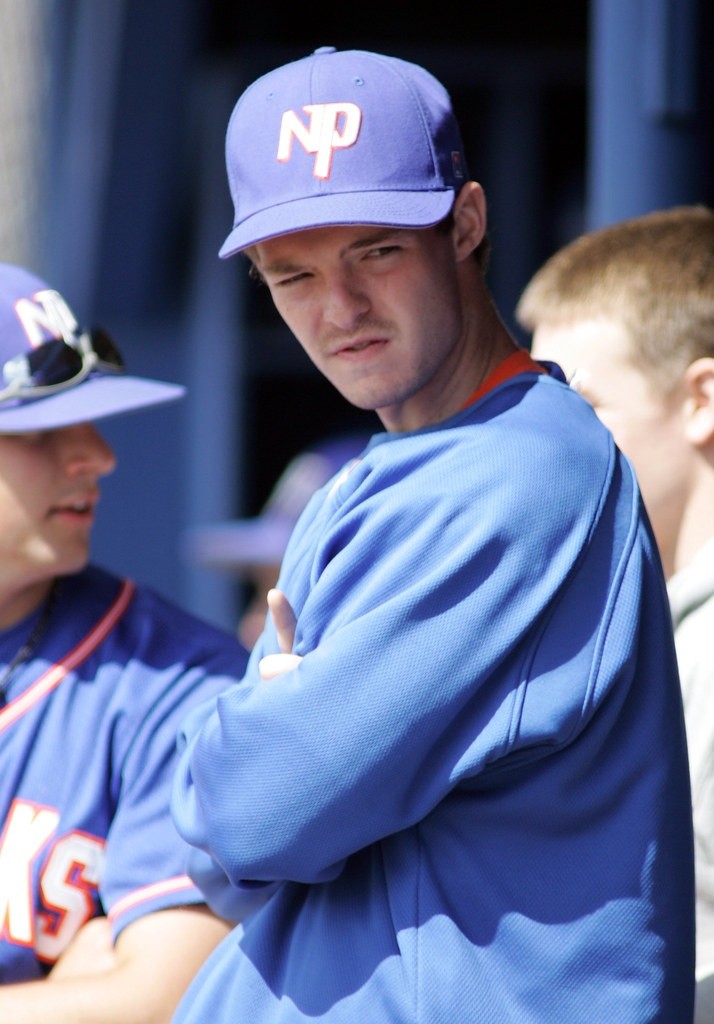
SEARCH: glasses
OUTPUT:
[0,324,124,403]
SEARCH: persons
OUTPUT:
[162,46,700,1024]
[0,210,713,1024]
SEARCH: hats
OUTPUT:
[218,45,466,260]
[0,264,188,436]
